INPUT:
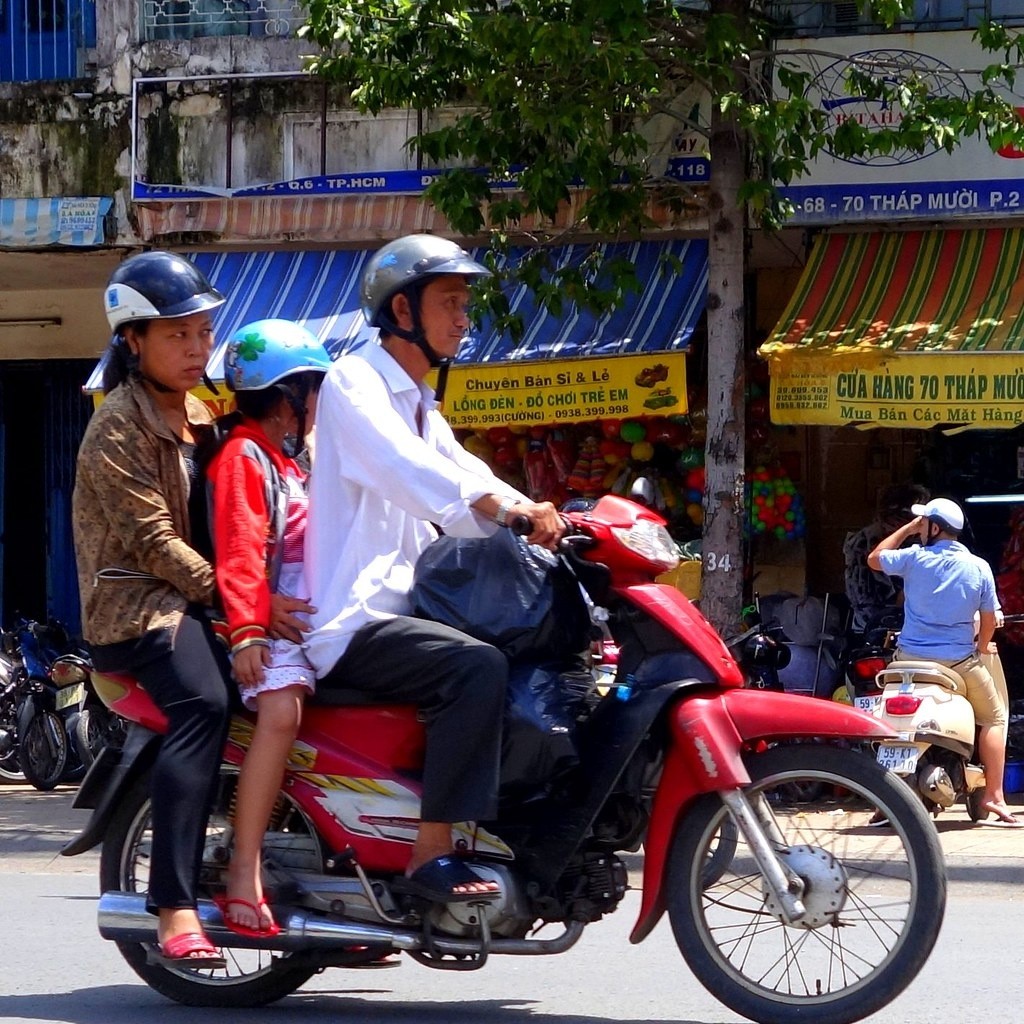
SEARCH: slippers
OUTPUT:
[976,812,1023,829]
[211,890,278,939]
[147,930,227,969]
[391,853,502,903]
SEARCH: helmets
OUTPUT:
[360,233,495,330]
[105,251,227,336]
[225,318,333,392]
[911,498,965,536]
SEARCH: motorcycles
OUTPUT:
[739,592,1023,823]
[0,599,133,788]
[62,477,951,1024]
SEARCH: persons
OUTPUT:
[292,228,608,911]
[865,498,1023,833]
[67,248,324,975]
[201,312,329,942]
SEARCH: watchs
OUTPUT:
[494,495,522,526]
